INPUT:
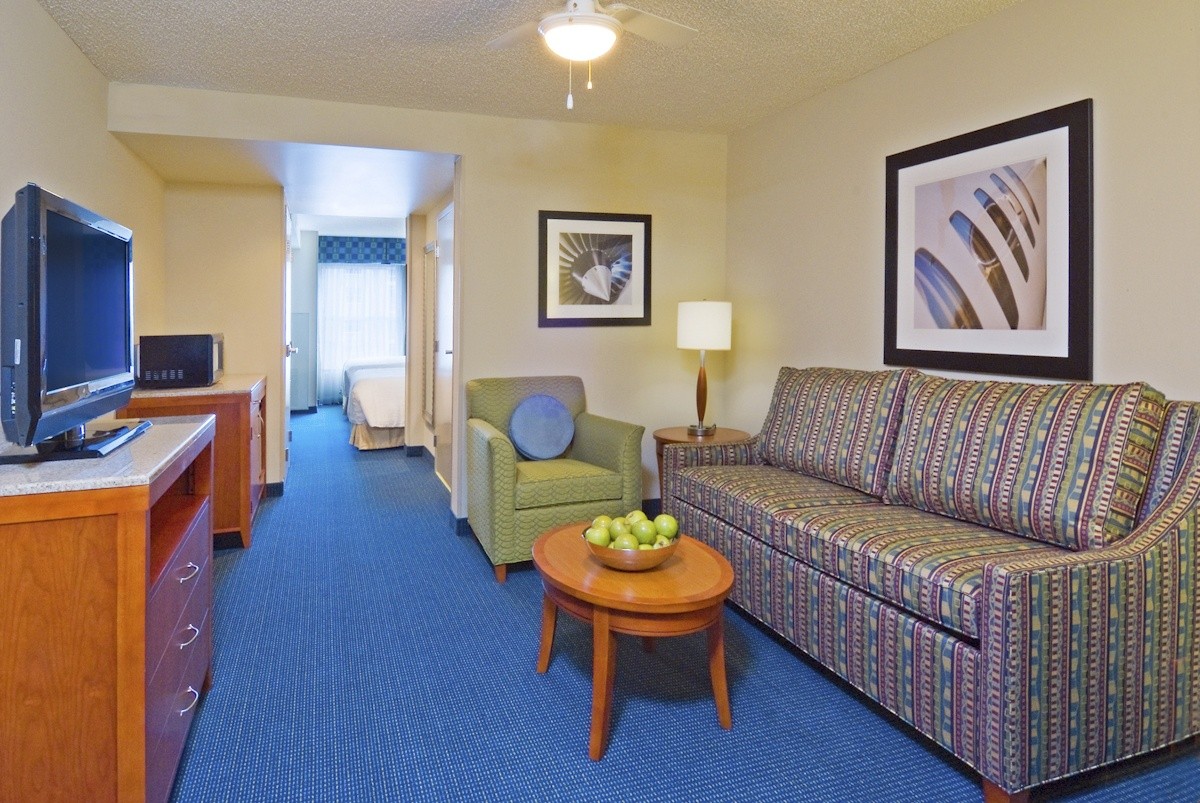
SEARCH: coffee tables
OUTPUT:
[534,520,735,758]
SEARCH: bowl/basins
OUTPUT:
[581,526,681,571]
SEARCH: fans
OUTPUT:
[479,0,700,58]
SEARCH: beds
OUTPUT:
[343,361,406,450]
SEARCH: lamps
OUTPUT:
[676,300,735,437]
[538,12,623,110]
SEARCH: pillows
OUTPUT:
[510,394,575,461]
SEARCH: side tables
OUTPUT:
[654,428,747,501]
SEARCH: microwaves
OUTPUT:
[140,333,224,389]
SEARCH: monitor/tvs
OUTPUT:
[0,185,152,466]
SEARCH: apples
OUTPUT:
[586,510,678,550]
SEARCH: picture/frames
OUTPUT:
[883,98,1095,382]
[540,209,651,328]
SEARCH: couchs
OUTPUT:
[466,375,644,582]
[661,365,1200,803]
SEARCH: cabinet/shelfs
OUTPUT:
[0,414,215,803]
[127,375,267,547]
